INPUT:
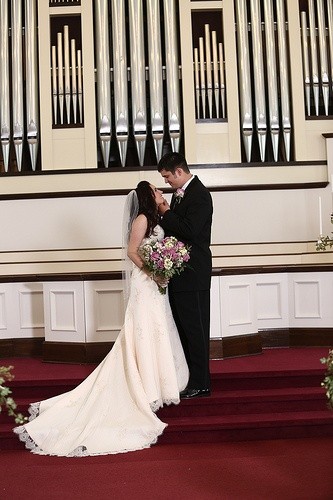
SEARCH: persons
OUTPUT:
[14,181,190,457]
[158,152,213,399]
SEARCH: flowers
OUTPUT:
[174,188,185,204]
[139,236,192,294]
